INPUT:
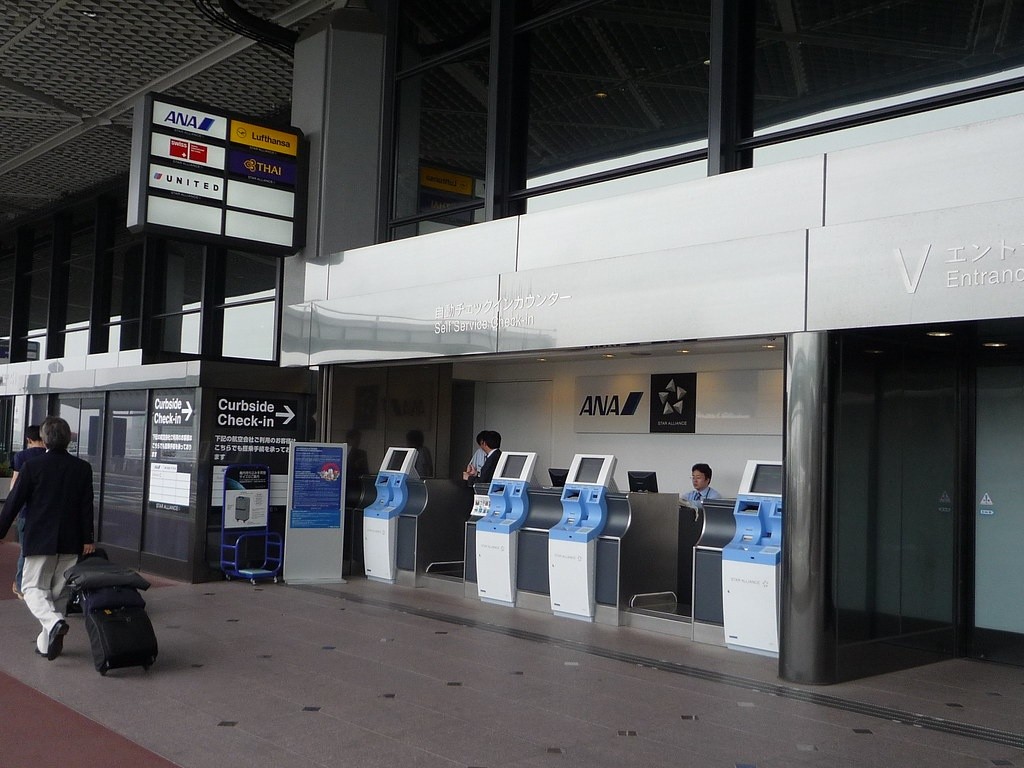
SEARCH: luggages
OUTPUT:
[78,548,158,676]
[65,596,83,617]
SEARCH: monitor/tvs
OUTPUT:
[386,450,408,471]
[501,455,528,478]
[628,471,658,493]
[575,458,604,483]
[750,464,782,494]
[548,468,569,487]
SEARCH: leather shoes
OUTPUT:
[48,619,69,661]
[35,648,48,657]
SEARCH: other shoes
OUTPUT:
[12,582,24,601]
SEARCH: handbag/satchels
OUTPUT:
[82,584,146,618]
[63,557,151,592]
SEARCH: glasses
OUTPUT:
[690,475,701,479]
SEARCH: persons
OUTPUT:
[344,429,369,474]
[9,425,46,600]
[0,416,96,660]
[681,464,722,508]
[394,429,433,476]
[463,431,502,485]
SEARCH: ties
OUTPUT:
[696,493,700,500]
